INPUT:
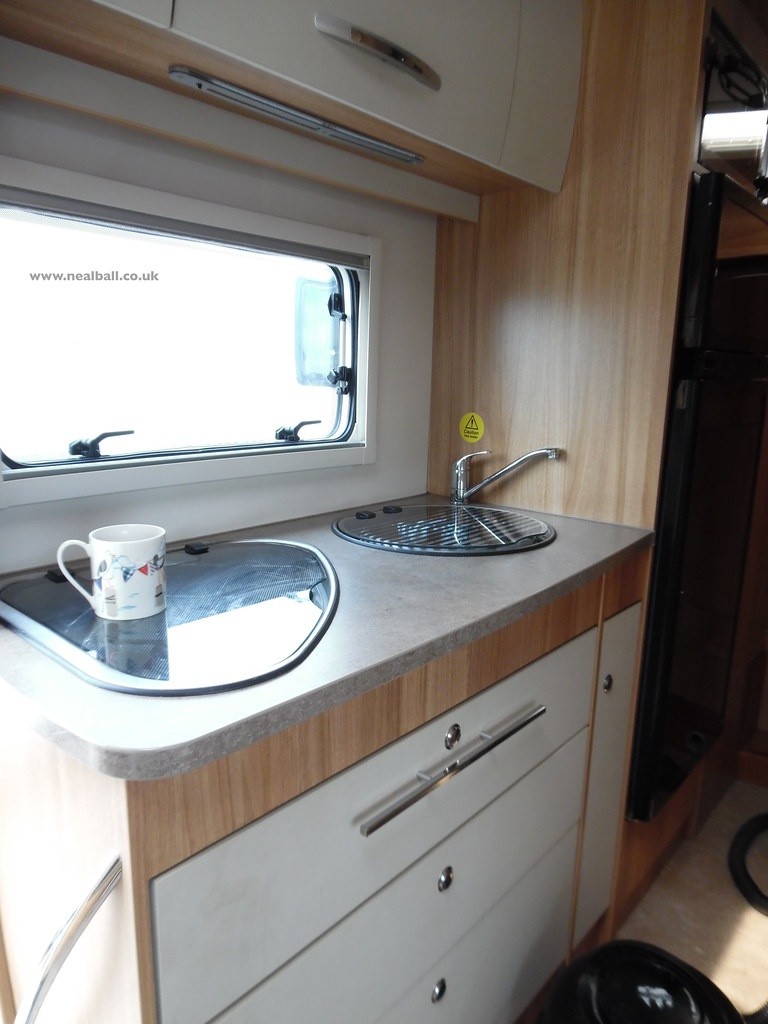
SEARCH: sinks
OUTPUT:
[333,503,558,556]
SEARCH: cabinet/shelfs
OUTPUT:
[0,0,584,221]
[0,548,652,1024]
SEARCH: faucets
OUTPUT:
[451,447,562,505]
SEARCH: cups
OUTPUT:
[56,524,168,621]
